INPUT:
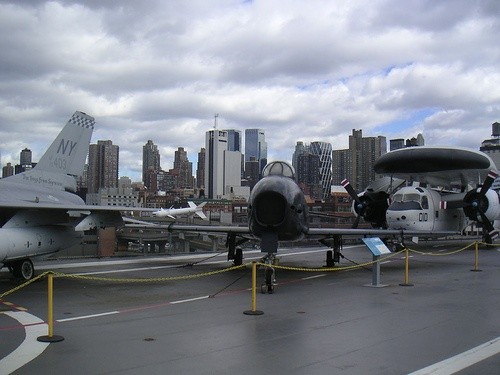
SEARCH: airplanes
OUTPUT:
[153,200,207,220]
[0,111,160,280]
[340,146,499,245]
[121,162,464,295]
